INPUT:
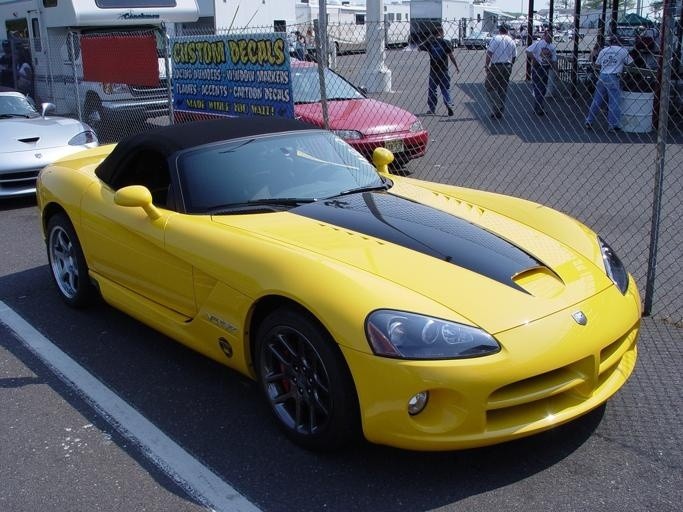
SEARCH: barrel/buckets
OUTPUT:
[619,89,656,134]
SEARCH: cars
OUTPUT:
[463,31,496,50]
[511,29,586,47]
[173,60,429,169]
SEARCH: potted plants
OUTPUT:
[618,55,658,134]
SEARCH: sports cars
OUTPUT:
[33,114,641,454]
[0,90,98,200]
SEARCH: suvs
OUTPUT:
[605,26,654,50]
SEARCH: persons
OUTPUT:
[485,23,517,119]
[417,22,459,116]
[288,29,317,63]
[585,25,679,133]
[0,39,34,99]
[519,24,557,46]
[524,29,558,117]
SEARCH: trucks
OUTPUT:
[0,0,200,131]
[166,0,335,69]
[294,0,393,56]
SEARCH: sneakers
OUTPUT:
[448,107,455,116]
[490,109,548,119]
[427,109,436,114]
[608,126,622,133]
[585,123,593,130]
